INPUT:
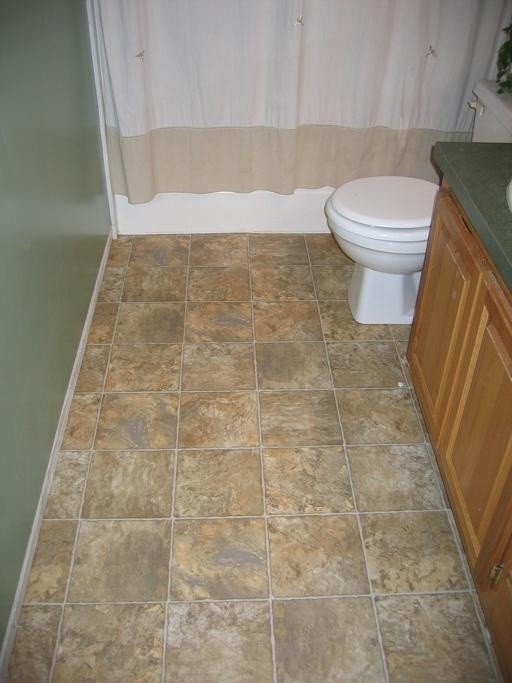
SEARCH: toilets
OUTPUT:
[324,79,512,326]
